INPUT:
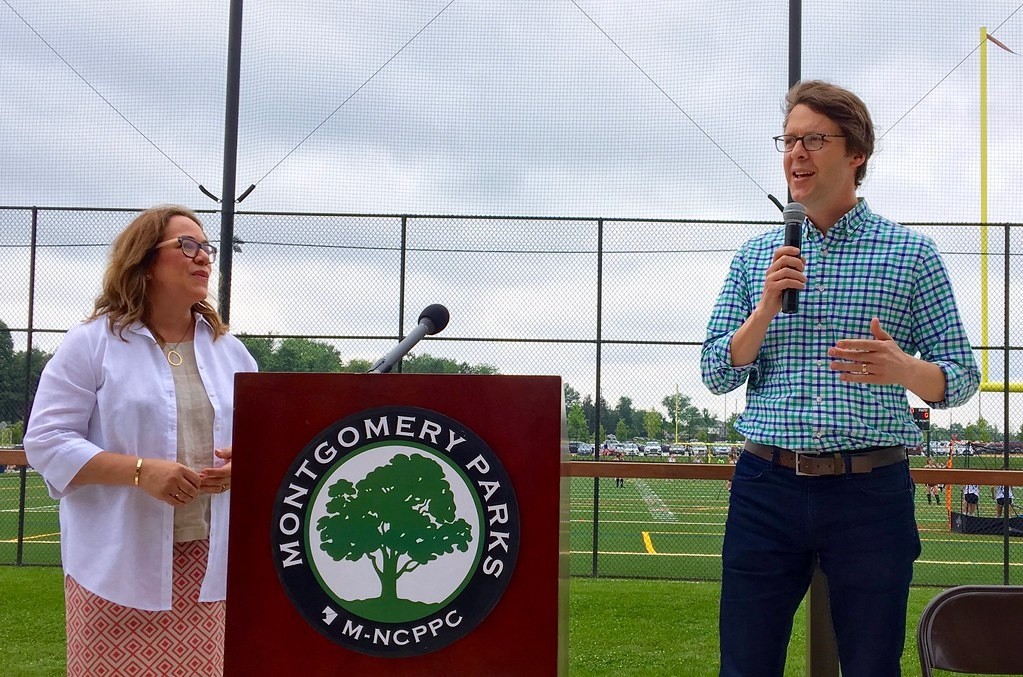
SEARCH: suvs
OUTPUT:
[644,442,661,456]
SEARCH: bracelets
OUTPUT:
[134,458,143,486]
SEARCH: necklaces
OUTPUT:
[166,318,193,366]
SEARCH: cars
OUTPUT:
[600,439,640,456]
[920,442,975,455]
[984,441,1023,454]
[662,444,671,452]
[691,445,707,456]
[669,444,685,454]
[712,446,730,455]
[568,440,595,454]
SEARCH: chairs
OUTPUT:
[916,584,1023,677]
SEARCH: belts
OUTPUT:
[744,438,907,477]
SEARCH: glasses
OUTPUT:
[772,133,846,151]
[154,237,217,264]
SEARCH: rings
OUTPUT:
[172,489,181,497]
[862,363,867,372]
[221,484,226,491]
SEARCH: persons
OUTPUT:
[666,454,676,482]
[693,456,703,463]
[603,441,608,456]
[963,484,980,516]
[701,81,981,677]
[616,452,625,488]
[23,204,258,677]
[726,445,742,492]
[991,466,1015,519]
[924,459,946,505]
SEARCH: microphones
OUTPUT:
[366,304,450,374]
[781,201,807,313]
